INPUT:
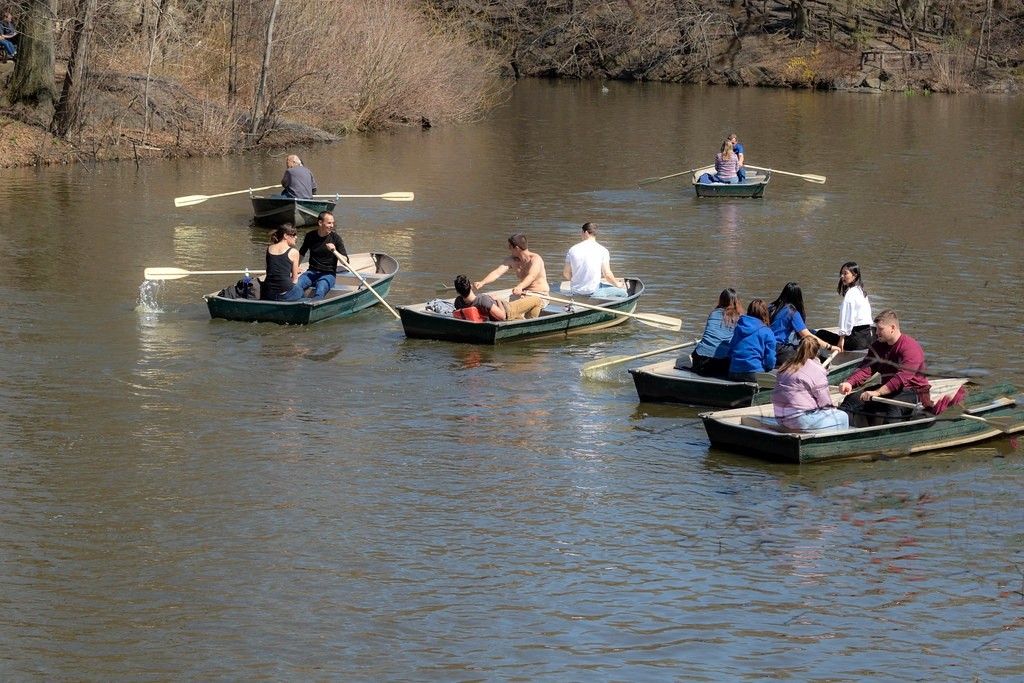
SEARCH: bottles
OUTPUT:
[244,268,251,283]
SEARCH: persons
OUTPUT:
[563,222,628,298]
[838,309,930,427]
[262,210,349,302]
[454,234,550,320]
[270,154,318,199]
[715,134,746,183]
[0,13,18,62]
[816,262,873,366]
[767,282,841,367]
[727,298,778,376]
[773,335,850,430]
[691,288,745,377]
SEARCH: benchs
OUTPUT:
[310,283,365,291]
[653,362,757,385]
[746,415,857,435]
[551,287,625,301]
[336,270,389,279]
[745,169,766,180]
[541,305,570,314]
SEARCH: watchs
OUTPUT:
[828,344,831,350]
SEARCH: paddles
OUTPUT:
[742,163,827,185]
[822,349,839,368]
[582,338,702,374]
[313,191,414,201]
[174,185,282,207]
[638,165,716,187]
[513,290,683,332]
[433,283,456,295]
[754,371,849,393]
[866,395,1014,433]
[144,267,267,280]
[325,243,400,319]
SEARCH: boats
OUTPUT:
[692,163,771,199]
[697,378,1024,465]
[201,252,399,326]
[628,327,870,409]
[395,277,644,346]
[249,195,337,229]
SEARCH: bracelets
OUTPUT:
[877,389,882,396]
[825,343,828,349]
